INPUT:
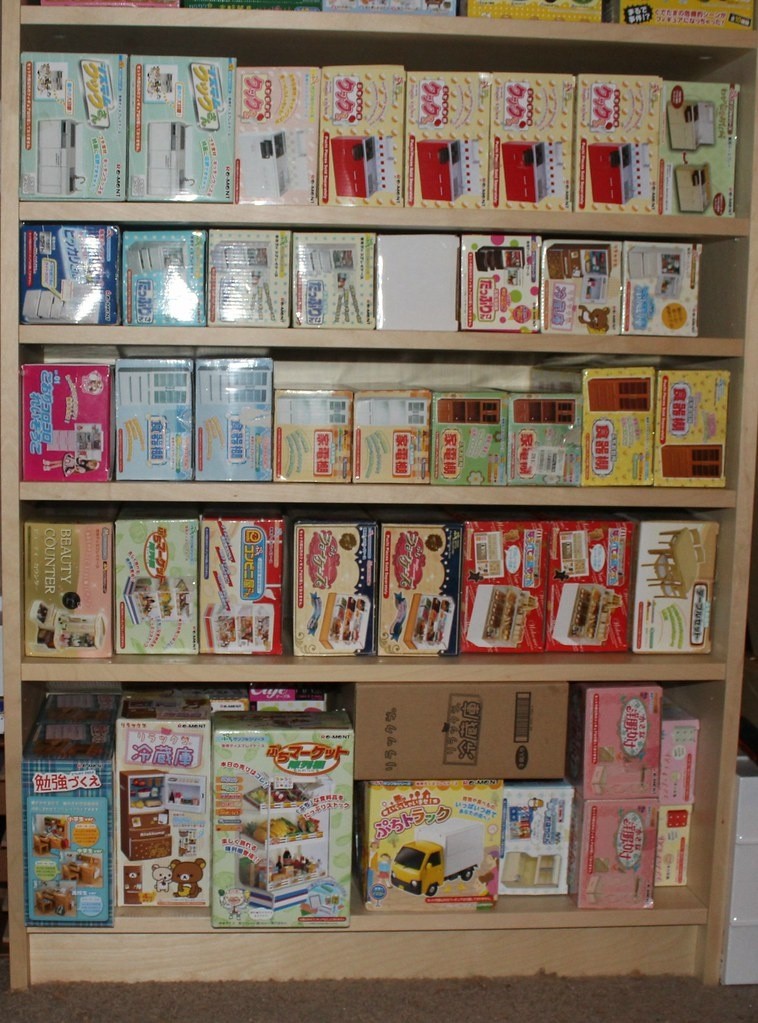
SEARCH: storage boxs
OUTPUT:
[20,0,754,928]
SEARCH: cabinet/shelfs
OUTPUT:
[0,0,758,988]
